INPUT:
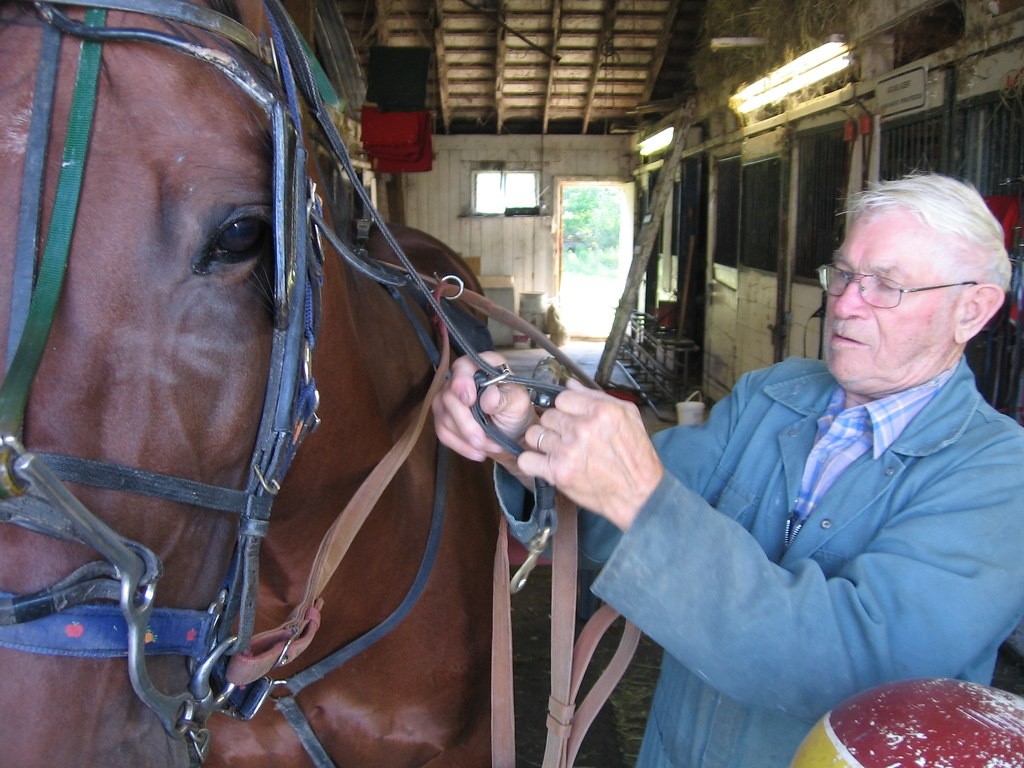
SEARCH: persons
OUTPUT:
[432,177,1024,768]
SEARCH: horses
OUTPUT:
[0,0,544,767]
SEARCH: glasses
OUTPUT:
[815,262,978,308]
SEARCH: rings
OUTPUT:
[537,431,546,450]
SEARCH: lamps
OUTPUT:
[635,125,675,156]
[727,32,847,103]
